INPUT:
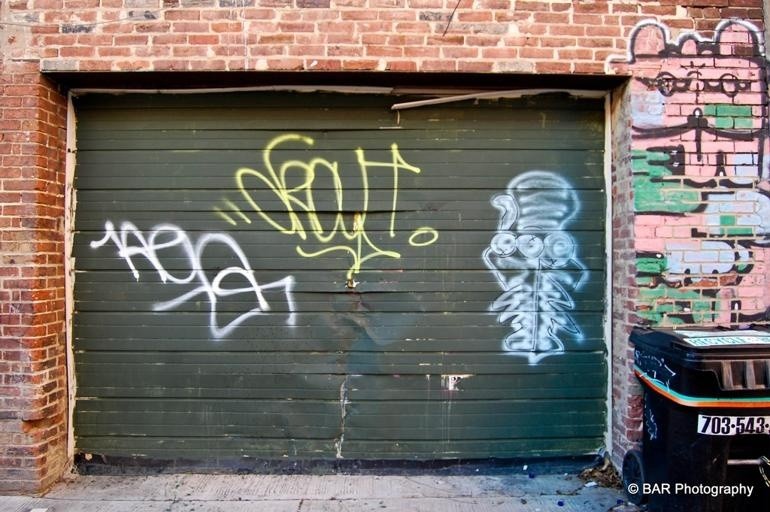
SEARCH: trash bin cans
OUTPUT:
[624,326,770,511]
